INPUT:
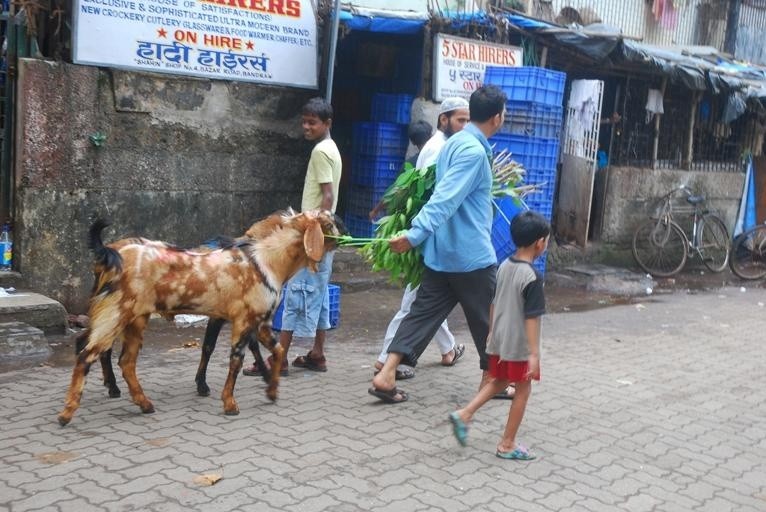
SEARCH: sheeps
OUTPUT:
[86,206,350,399]
[58,212,341,428]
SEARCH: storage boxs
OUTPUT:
[371,92,414,124]
[345,122,409,238]
[483,65,567,277]
[273,281,340,332]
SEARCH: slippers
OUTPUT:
[492,385,517,400]
[447,409,473,449]
[372,364,415,381]
[440,342,465,367]
[366,385,409,404]
[495,444,538,461]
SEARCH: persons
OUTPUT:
[368,84,517,404]
[449,210,551,459]
[368,116,433,224]
[375,96,465,381]
[243,96,343,378]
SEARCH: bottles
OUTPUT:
[0,222,13,270]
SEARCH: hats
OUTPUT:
[437,96,471,115]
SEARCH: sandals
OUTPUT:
[290,349,328,373]
[240,354,289,377]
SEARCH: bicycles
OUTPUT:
[616,184,730,276]
[731,215,766,282]
